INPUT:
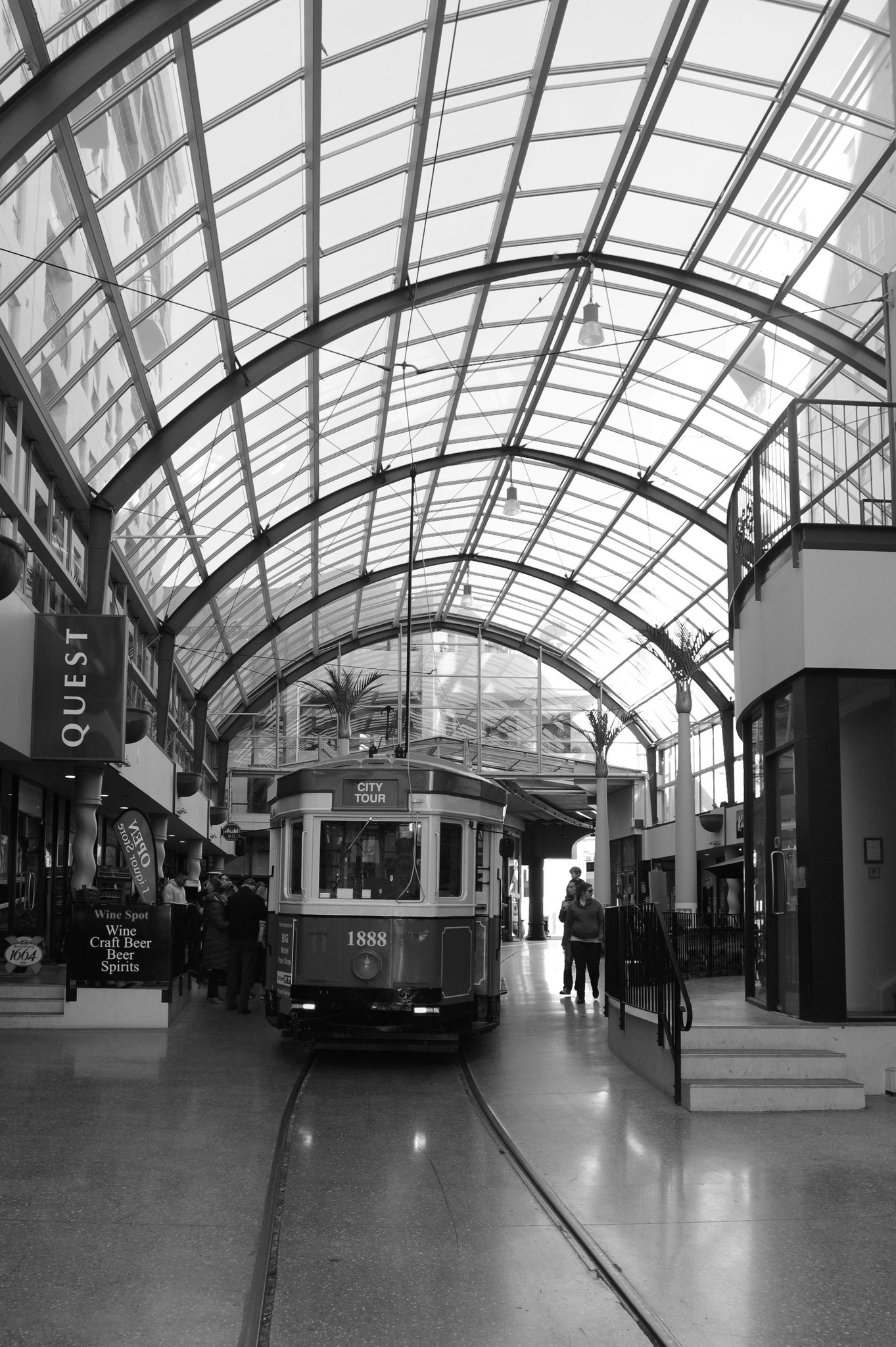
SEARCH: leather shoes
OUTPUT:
[206,996,226,1004]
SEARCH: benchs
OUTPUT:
[319,859,415,899]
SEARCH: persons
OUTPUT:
[558,879,581,995]
[162,869,187,906]
[569,867,585,883]
[389,837,416,899]
[197,874,267,1015]
[561,882,605,1003]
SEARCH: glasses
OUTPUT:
[584,889,594,894]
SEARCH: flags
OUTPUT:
[111,807,158,905]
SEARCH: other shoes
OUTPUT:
[218,979,226,984]
[592,986,599,998]
[199,978,204,982]
[563,908,567,911]
[577,995,585,1003]
[574,985,577,991]
[227,1004,237,1010]
[249,993,256,998]
[561,943,565,951]
[239,1009,251,1014]
[560,988,571,994]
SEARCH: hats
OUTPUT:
[244,879,256,886]
[210,878,222,889]
[256,882,265,888]
[219,881,234,893]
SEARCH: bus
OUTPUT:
[262,747,508,1059]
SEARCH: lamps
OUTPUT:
[578,257,608,348]
[459,556,474,608]
[501,454,523,518]
